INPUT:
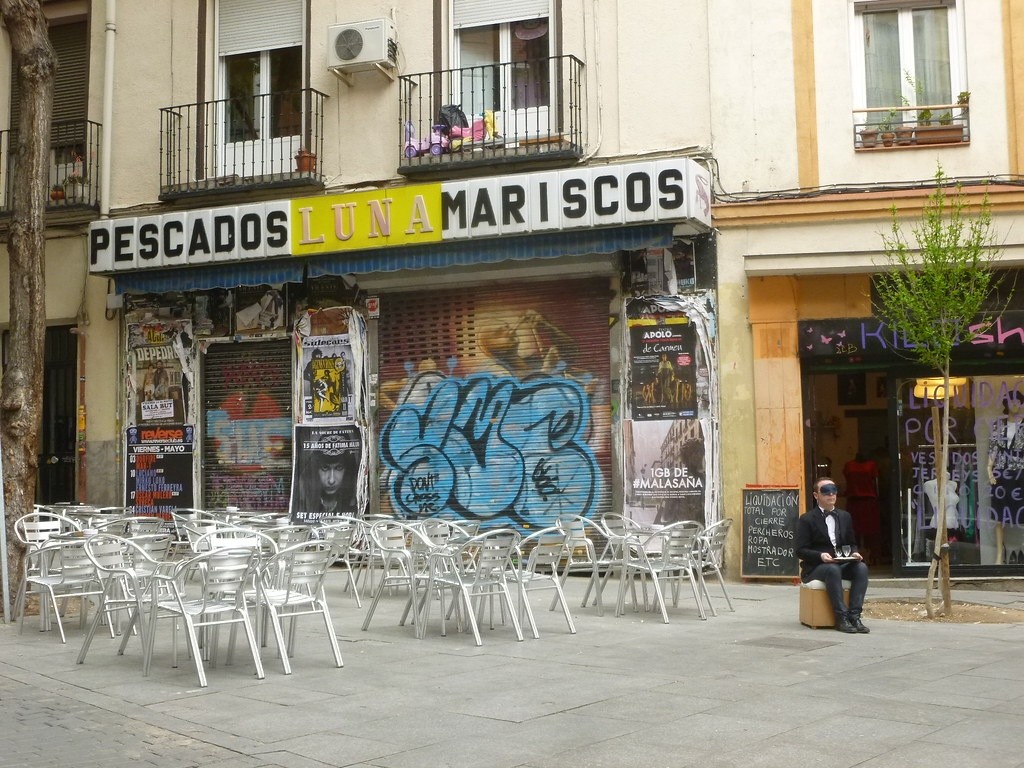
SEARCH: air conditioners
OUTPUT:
[325,16,397,74]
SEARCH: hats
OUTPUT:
[1002,390,1024,406]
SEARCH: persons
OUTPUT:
[643,353,692,403]
[843,453,880,536]
[794,477,872,634]
[988,390,1024,564]
[143,360,169,401]
[301,432,358,514]
[923,472,960,529]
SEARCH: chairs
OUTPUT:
[558,483,736,623]
[11,499,578,688]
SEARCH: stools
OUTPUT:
[799,580,853,630]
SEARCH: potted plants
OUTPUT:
[295,149,317,172]
[857,69,970,148]
[50,174,90,200]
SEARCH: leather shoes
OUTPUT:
[848,615,870,633]
[836,615,857,633]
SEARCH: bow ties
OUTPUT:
[823,509,839,519]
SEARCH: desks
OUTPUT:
[42,503,701,662]
